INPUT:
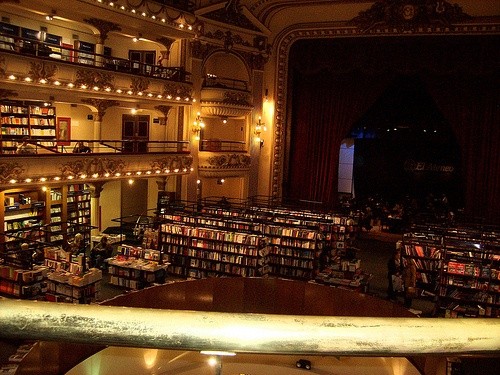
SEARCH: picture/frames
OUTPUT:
[57,117,71,147]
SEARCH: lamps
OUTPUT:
[46,11,57,20]
[134,33,143,41]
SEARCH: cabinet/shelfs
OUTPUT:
[0,100,57,154]
[1,184,500,375]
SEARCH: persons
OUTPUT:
[347,192,448,219]
[16,137,35,154]
[386,250,401,299]
[21,242,33,269]
[73,232,85,255]
[216,196,229,206]
[93,235,113,269]
[158,56,163,72]
[74,142,91,154]
[404,257,416,307]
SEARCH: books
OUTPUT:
[0,191,362,306]
[402,223,500,319]
[0,104,55,154]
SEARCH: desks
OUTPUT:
[90,233,126,249]
